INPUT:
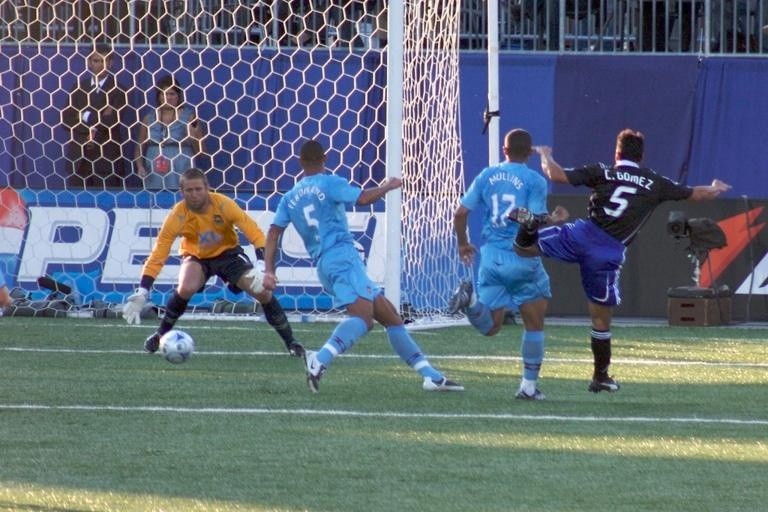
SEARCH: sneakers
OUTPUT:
[448,280,473,315]
[306,349,326,391]
[508,208,550,229]
[587,379,619,393]
[515,389,545,400]
[288,343,303,354]
[144,332,159,352]
[424,376,464,391]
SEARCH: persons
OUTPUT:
[445,128,571,401]
[507,128,734,393]
[133,75,207,190]
[261,140,465,395]
[121,167,306,358]
[59,43,129,188]
[0,269,13,317]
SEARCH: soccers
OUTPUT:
[159,329,194,364]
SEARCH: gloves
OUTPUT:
[244,261,267,294]
[121,288,148,327]
